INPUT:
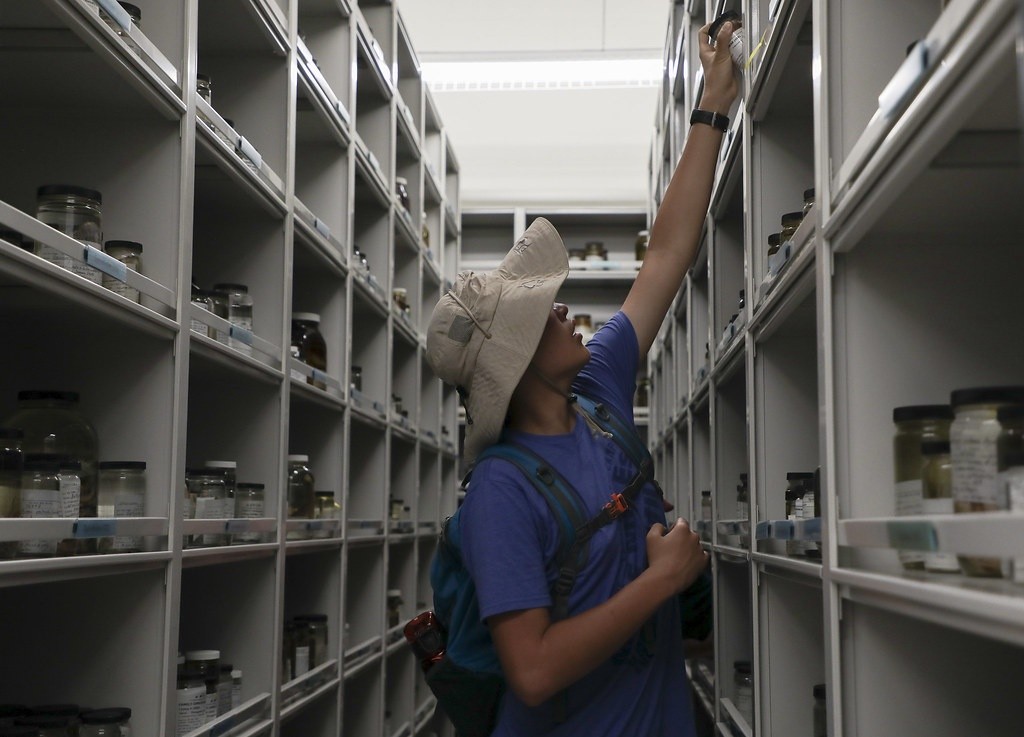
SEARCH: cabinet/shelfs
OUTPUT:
[1,0,1024,737]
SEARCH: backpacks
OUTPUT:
[429,394,655,737]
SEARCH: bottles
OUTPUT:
[176,644,243,733]
[354,244,370,289]
[351,366,361,407]
[396,175,411,212]
[721,289,744,331]
[0,702,133,737]
[292,309,328,391]
[183,461,266,549]
[568,239,609,273]
[784,465,821,561]
[287,453,339,542]
[0,184,145,307]
[887,383,1024,581]
[191,276,254,347]
[196,73,212,107]
[0,387,149,557]
[699,490,712,541]
[735,473,750,547]
[635,230,648,270]
[732,659,753,726]
[281,615,328,686]
[98,1,141,58]
[707,9,742,73]
[390,494,413,534]
[393,287,411,323]
[811,682,827,737]
[765,188,813,269]
[574,315,591,334]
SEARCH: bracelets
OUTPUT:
[690,109,729,132]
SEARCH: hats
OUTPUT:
[425,218,568,469]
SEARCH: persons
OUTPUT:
[427,22,741,737]
